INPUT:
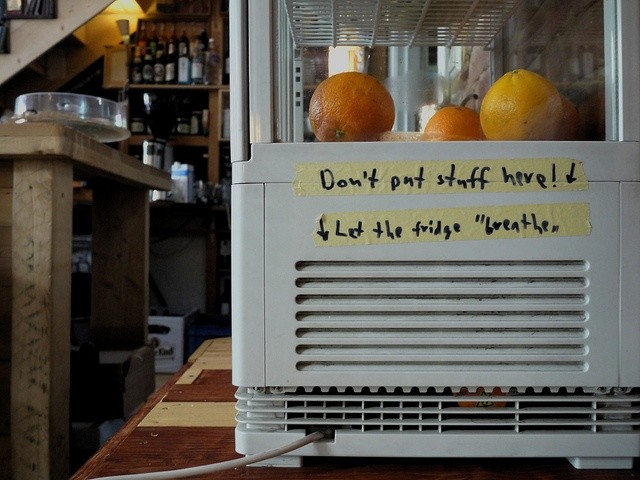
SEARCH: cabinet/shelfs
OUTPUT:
[127,11,229,187]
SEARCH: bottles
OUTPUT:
[199,23,209,51]
[169,24,179,53]
[204,39,220,85]
[178,26,190,57]
[165,40,177,84]
[143,47,154,84]
[148,24,159,53]
[131,44,142,84]
[157,23,169,49]
[137,20,148,56]
[177,47,192,85]
[192,41,204,83]
[154,40,165,84]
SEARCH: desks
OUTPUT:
[0,122,171,480]
